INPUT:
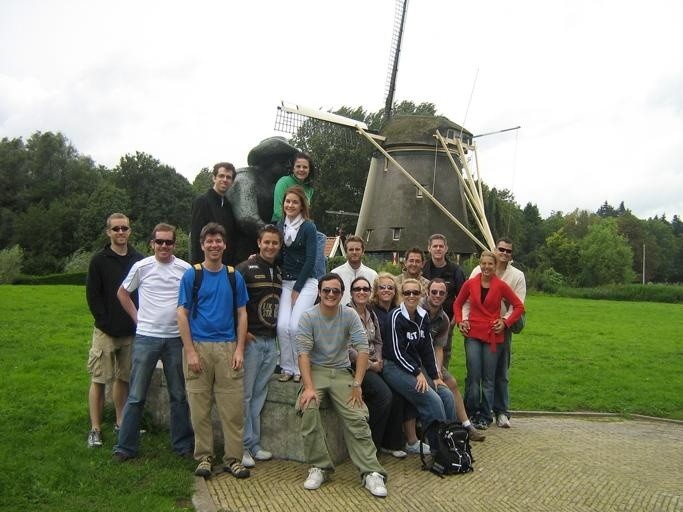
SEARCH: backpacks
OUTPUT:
[420,416,476,478]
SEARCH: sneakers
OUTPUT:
[304,467,330,489]
[178,449,196,464]
[293,374,302,382]
[225,460,250,479]
[406,439,433,455]
[238,453,257,468]
[113,425,148,436]
[466,424,485,441]
[89,430,104,449]
[194,457,213,477]
[476,414,491,429]
[496,415,512,429]
[277,371,292,382]
[254,448,272,461]
[110,450,131,467]
[383,447,407,458]
[363,471,388,498]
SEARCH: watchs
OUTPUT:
[351,381,363,386]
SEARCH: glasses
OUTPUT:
[111,226,131,232]
[431,290,447,295]
[352,287,371,292]
[378,285,395,290]
[403,290,421,296]
[155,238,174,245]
[322,287,342,295]
[499,247,513,254]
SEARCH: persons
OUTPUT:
[368,272,431,454]
[394,247,432,298]
[272,153,314,225]
[294,273,388,497]
[113,223,196,462]
[385,278,458,451]
[463,238,526,428]
[176,223,250,477]
[234,225,283,467]
[330,236,378,307]
[192,162,243,265]
[86,213,147,447]
[454,251,524,429]
[274,185,326,382]
[421,234,466,371]
[344,277,406,457]
